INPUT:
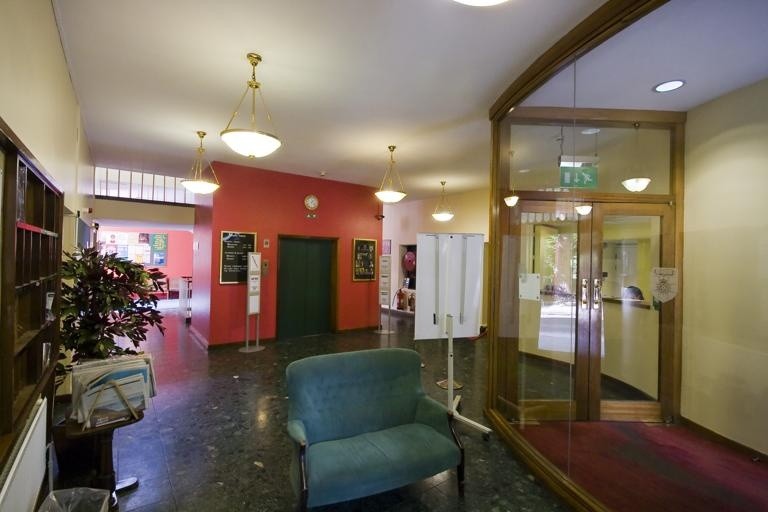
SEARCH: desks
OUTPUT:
[56,412,146,496]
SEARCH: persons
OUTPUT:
[624,285,644,300]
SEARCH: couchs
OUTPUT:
[280,345,469,511]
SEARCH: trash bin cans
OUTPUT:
[37,487,110,512]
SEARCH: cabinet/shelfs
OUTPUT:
[0,117,67,492]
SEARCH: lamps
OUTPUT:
[179,52,282,194]
[374,145,406,201]
[620,121,653,193]
[503,150,521,208]
[431,180,453,222]
